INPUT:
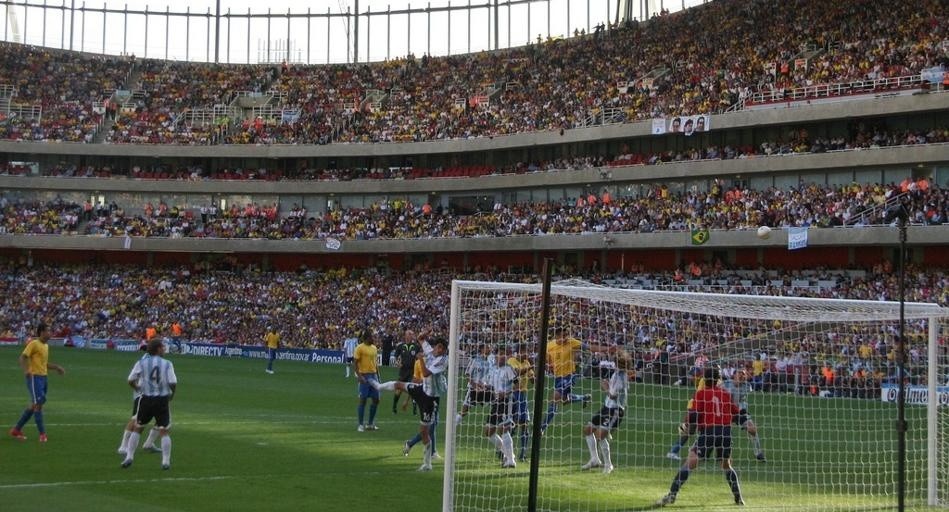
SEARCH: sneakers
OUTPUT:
[368,378,379,388]
[581,458,601,470]
[121,457,133,468]
[656,494,676,506]
[501,454,515,467]
[39,432,47,442]
[403,440,410,456]
[265,368,270,373]
[503,461,516,468]
[117,446,128,456]
[142,443,162,452]
[269,371,274,374]
[666,453,680,460]
[416,464,432,472]
[583,394,591,408]
[756,454,766,463]
[357,425,364,432]
[365,424,379,430]
[603,464,614,474]
[161,464,169,469]
[9,427,27,440]
[736,501,745,506]
[431,452,441,459]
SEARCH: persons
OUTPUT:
[402,398,445,462]
[702,253,737,380]
[496,10,665,137]
[685,367,723,409]
[719,366,767,463]
[368,260,452,349]
[456,345,491,441]
[341,331,359,379]
[299,265,368,343]
[773,120,947,155]
[0,256,265,353]
[490,254,543,358]
[500,142,669,173]
[738,257,949,397]
[580,344,630,474]
[540,328,590,435]
[546,260,701,386]
[367,342,446,471]
[119,338,176,469]
[661,368,757,506]
[352,329,381,432]
[660,357,711,458]
[485,350,516,470]
[267,262,297,332]
[409,349,439,419]
[671,137,771,162]
[507,344,533,463]
[393,328,418,417]
[452,256,490,358]
[262,327,283,375]
[669,114,710,135]
[280,333,345,352]
[119,346,164,456]
[668,1,948,111]
[11,324,64,442]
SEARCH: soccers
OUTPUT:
[757,226,772,239]
